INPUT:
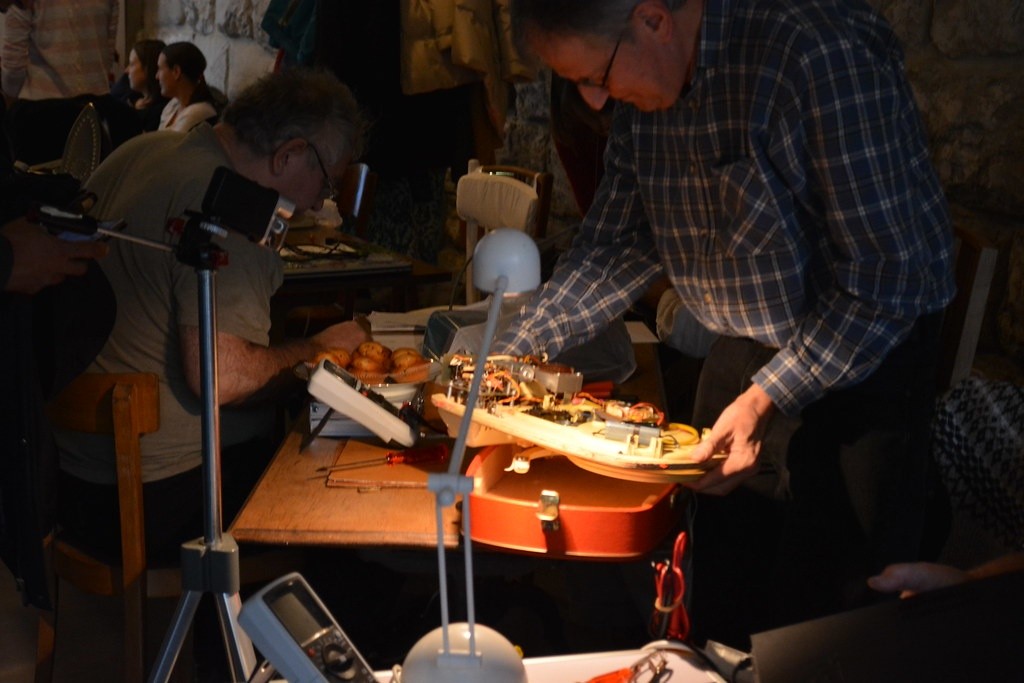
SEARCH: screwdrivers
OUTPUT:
[317,443,448,473]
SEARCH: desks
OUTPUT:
[368,639,726,683]
[279,228,437,293]
[4,215,454,339]
[227,412,460,548]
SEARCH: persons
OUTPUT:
[423,0,959,654]
[867,554,1024,604]
[0,0,369,571]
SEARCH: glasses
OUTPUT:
[575,1,669,90]
[307,142,346,198]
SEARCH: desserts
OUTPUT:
[306,341,431,385]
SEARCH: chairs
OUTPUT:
[456,159,552,305]
[33,371,302,683]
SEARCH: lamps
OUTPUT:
[399,229,540,683]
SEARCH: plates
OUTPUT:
[567,445,729,483]
[293,356,442,393]
